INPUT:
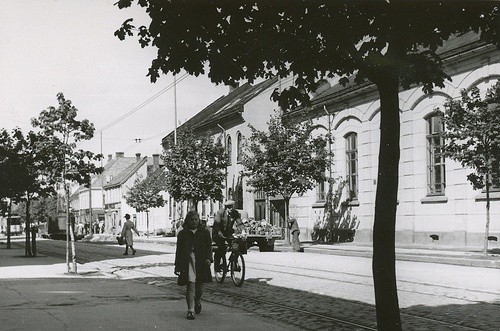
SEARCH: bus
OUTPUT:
[0,212,23,236]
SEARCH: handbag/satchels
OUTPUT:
[177,263,188,285]
[118,237,124,245]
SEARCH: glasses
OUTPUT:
[225,205,233,208]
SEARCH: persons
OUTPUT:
[74,219,105,235]
[212,200,250,272]
[287,216,301,252]
[120,214,141,255]
[174,211,213,320]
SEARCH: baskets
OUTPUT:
[231,239,247,254]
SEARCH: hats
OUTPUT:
[223,200,235,205]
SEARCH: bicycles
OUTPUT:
[213,236,246,287]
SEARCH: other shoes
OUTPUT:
[132,250,136,255]
[123,251,128,255]
[194,296,202,314]
[214,260,220,270]
[187,312,195,320]
[230,261,237,271]
